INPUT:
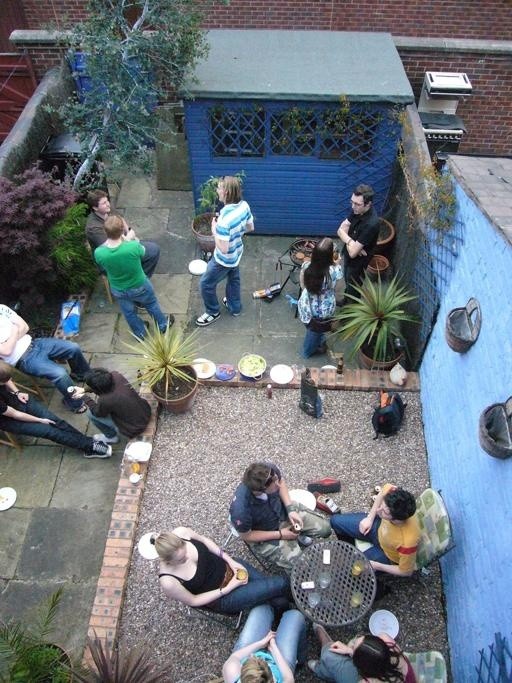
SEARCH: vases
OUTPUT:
[367,255,389,280]
[374,216,395,254]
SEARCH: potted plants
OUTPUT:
[332,261,424,369]
[191,168,247,250]
[119,312,216,414]
[1,581,75,682]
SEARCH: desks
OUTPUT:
[291,539,376,627]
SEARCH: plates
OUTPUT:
[215,363,237,381]
[0,485,17,512]
[269,363,294,385]
[190,357,216,380]
[368,609,399,642]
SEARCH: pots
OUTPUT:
[238,351,267,380]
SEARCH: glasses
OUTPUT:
[259,467,275,491]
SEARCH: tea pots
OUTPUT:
[119,455,141,477]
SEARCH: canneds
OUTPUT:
[374,486,381,493]
[370,496,377,507]
[67,386,75,397]
[293,522,301,533]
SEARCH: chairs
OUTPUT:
[348,636,449,682]
[86,240,115,303]
[355,486,457,586]
[11,358,67,402]
[0,428,23,452]
[224,514,276,571]
[186,607,243,629]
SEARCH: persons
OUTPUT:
[229,462,332,579]
[222,604,307,683]
[307,622,416,683]
[71,367,151,444]
[195,176,254,326]
[150,526,297,614]
[94,215,175,343]
[298,238,346,359]
[329,483,420,578]
[0,303,91,414]
[0,359,112,458]
[85,189,161,310]
[337,184,380,323]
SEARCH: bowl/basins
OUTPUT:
[125,441,152,463]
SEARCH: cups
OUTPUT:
[292,362,305,380]
[317,572,332,588]
[349,591,365,609]
[350,559,365,575]
[237,568,248,579]
[306,591,322,609]
[129,472,144,486]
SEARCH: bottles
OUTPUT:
[266,281,281,296]
[335,356,344,379]
[333,247,338,260]
[215,212,219,222]
[252,289,267,298]
[316,368,328,400]
[266,384,273,398]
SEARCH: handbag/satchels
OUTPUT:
[307,316,335,332]
[372,392,404,433]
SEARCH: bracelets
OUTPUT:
[286,505,295,513]
[220,586,223,596]
[15,391,20,395]
[347,238,352,245]
[279,530,282,540]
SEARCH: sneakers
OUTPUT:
[84,433,119,458]
[308,479,341,514]
[196,310,220,325]
[160,313,174,333]
[308,660,318,670]
[222,295,240,315]
[312,622,333,646]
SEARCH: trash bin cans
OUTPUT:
[38,132,110,217]
[153,105,192,190]
